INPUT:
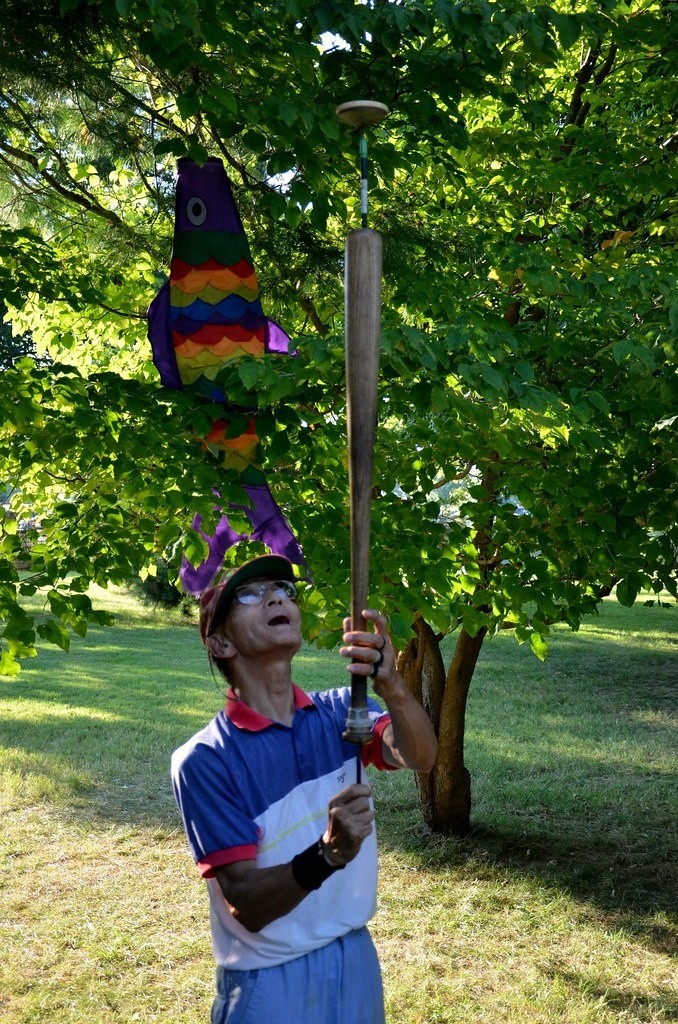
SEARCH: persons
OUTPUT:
[170,554,437,1024]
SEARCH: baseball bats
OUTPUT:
[341,227,384,745]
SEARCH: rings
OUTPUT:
[369,636,386,678]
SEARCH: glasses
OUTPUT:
[234,580,299,606]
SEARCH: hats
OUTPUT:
[199,552,296,644]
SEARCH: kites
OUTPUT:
[147,156,310,593]
